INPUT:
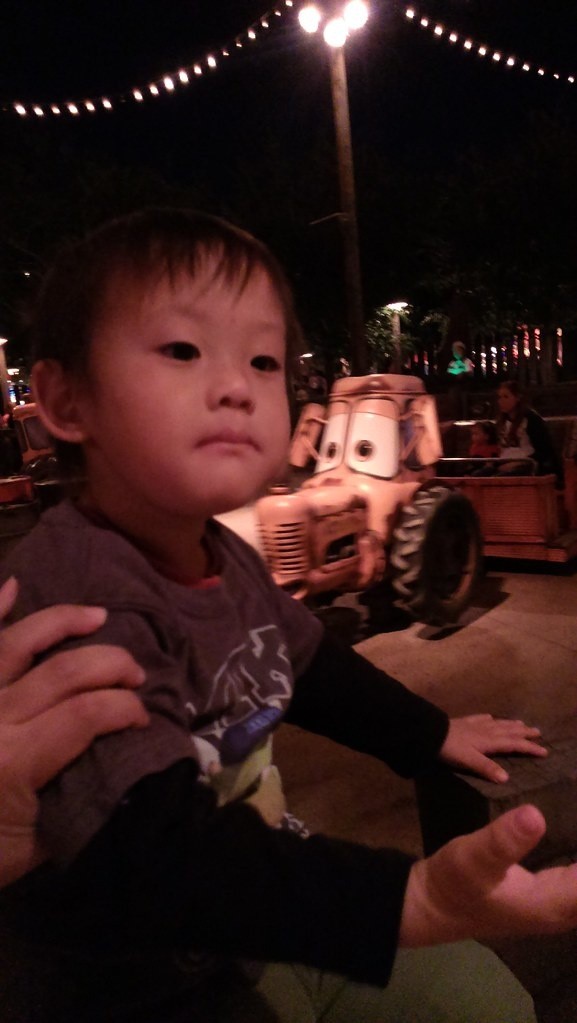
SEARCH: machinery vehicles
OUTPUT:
[0,401,79,512]
[211,372,481,637]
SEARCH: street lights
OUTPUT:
[384,296,409,372]
[299,0,374,366]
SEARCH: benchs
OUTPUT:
[438,416,577,544]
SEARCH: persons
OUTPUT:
[0,204,577,1023]
